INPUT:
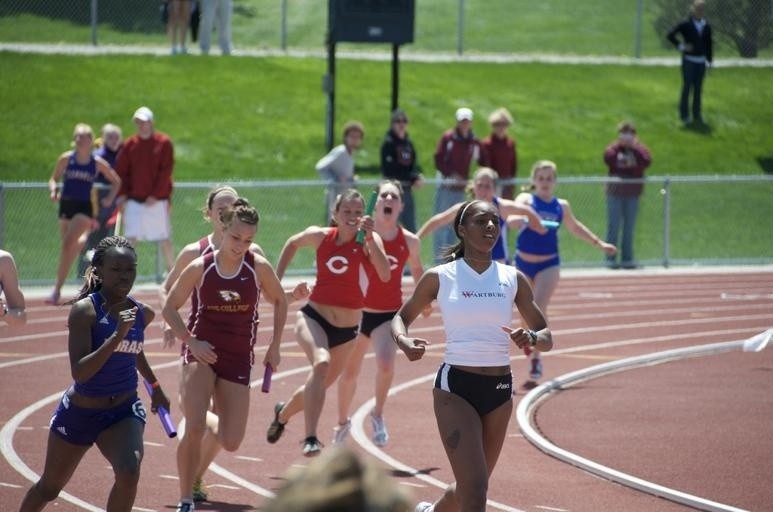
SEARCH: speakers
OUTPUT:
[329,0,414,43]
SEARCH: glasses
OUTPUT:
[394,119,407,125]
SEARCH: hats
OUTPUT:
[132,106,152,123]
[391,109,405,119]
[456,107,472,122]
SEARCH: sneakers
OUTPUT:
[266,403,286,443]
[176,497,194,512]
[371,409,388,445]
[302,439,321,456]
[332,418,351,444]
[529,360,542,379]
[194,478,207,502]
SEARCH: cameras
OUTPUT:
[621,135,633,143]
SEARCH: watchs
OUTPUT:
[527,329,537,341]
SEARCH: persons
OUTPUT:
[316,121,366,197]
[0,250,28,328]
[50,106,175,305]
[267,179,433,459]
[21,236,171,512]
[667,1,712,129]
[391,199,553,512]
[417,167,548,267]
[168,0,191,54]
[602,122,652,269]
[159,184,311,512]
[504,160,618,378]
[377,110,425,235]
[198,0,233,56]
[432,107,519,268]
